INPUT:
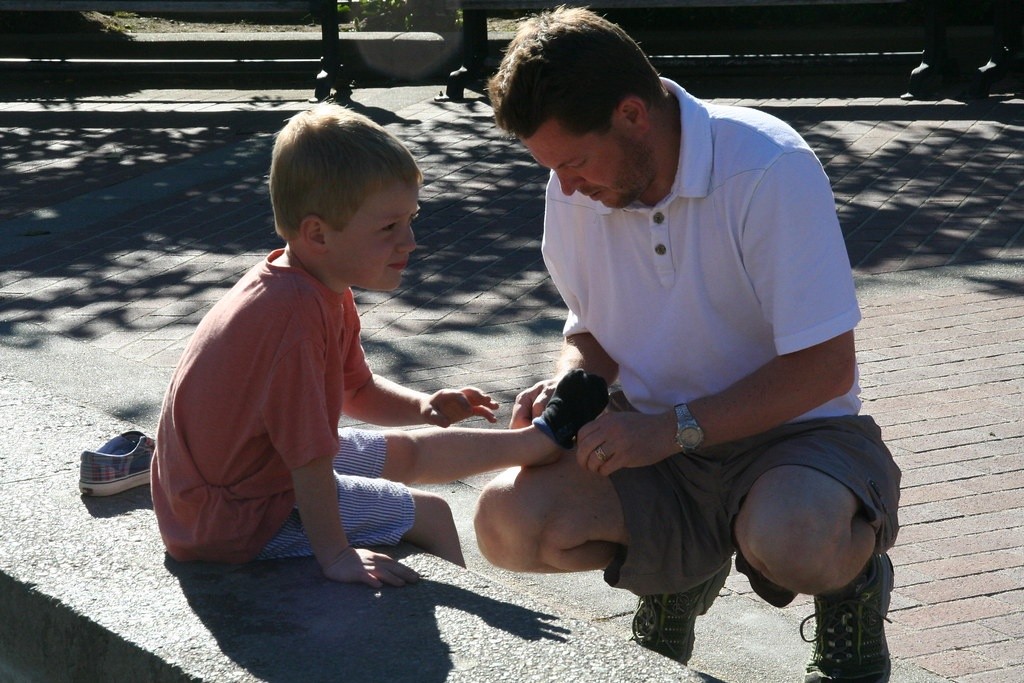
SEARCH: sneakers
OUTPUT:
[799,553,894,683]
[630,557,731,668]
[78,430,156,497]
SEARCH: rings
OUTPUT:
[593,447,608,464]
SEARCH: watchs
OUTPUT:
[673,402,704,456]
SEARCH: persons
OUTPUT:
[148,108,610,590]
[473,5,901,683]
